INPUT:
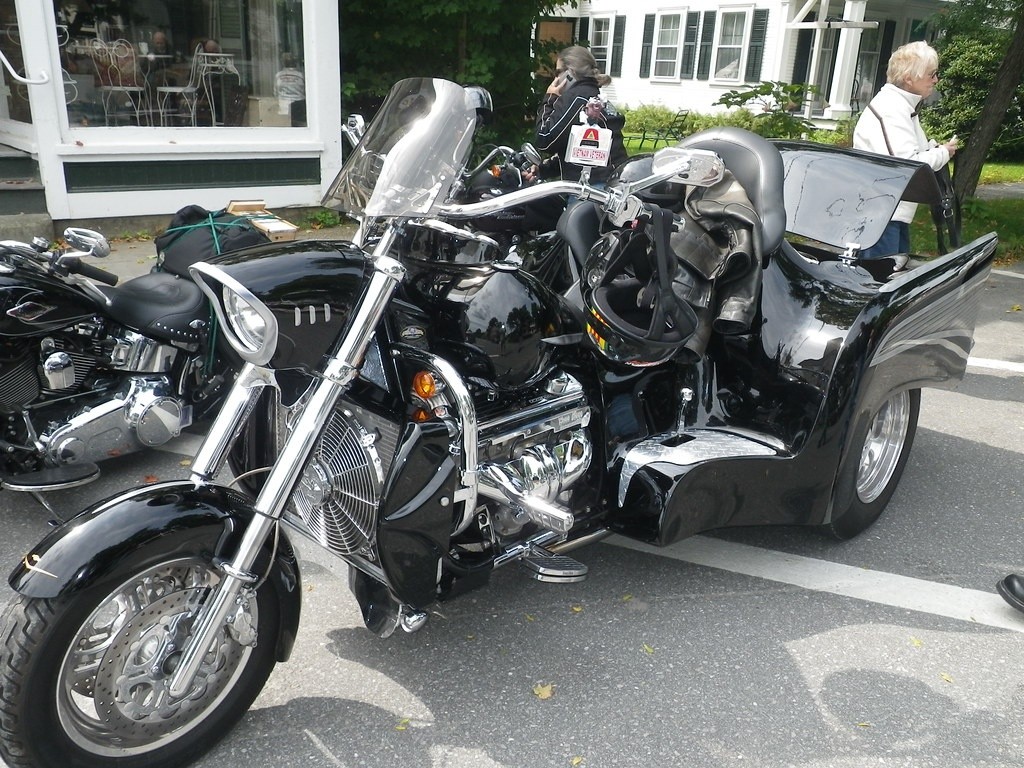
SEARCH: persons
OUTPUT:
[518,47,630,208]
[272,51,304,115]
[849,41,960,262]
[94,24,224,126]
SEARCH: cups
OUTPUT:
[138,42,148,54]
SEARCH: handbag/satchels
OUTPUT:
[929,164,963,255]
[155,206,260,280]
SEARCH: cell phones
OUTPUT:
[555,70,577,95]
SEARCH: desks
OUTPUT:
[136,55,174,127]
[196,52,241,127]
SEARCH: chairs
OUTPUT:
[91,37,150,127]
[638,109,689,151]
[850,79,861,119]
[157,42,205,127]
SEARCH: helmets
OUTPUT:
[584,279,698,369]
[457,84,492,126]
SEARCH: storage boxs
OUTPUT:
[234,208,300,243]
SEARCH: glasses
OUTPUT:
[554,68,570,77]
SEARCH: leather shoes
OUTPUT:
[996,575,1024,613]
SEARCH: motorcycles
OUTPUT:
[0,72,1000,768]
[0,199,294,533]
